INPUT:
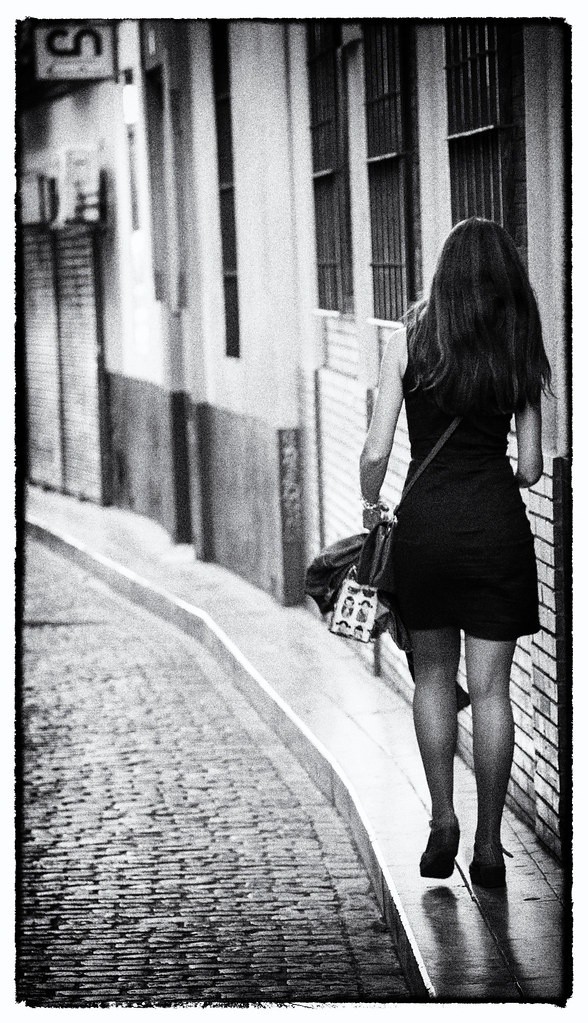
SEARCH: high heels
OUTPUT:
[468,843,513,888]
[420,811,460,878]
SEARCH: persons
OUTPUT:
[360,218,553,882]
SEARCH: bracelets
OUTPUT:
[360,496,383,513]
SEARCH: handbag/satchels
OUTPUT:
[327,565,380,643]
[355,519,396,587]
